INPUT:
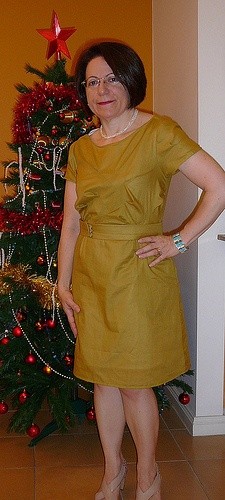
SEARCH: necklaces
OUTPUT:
[99,108,139,140]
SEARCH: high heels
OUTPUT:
[94,457,128,500]
[135,460,160,500]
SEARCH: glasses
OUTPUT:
[81,72,120,88]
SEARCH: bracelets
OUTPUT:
[172,231,190,255]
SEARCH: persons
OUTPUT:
[55,38,225,500]
